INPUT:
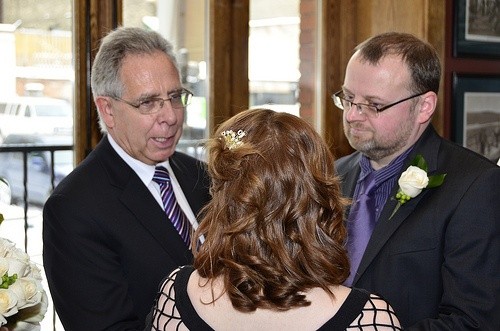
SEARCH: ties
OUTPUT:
[152,165,202,255]
[341,175,379,291]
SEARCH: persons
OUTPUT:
[147,107,404,331]
[43,26,212,331]
[332,32,500,330]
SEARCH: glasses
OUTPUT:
[332,89,434,118]
[103,87,194,111]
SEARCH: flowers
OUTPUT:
[0,237,49,331]
[388,153,447,221]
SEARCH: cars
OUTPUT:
[0,97,75,207]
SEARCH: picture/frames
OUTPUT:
[452,0,500,60]
[451,71,500,168]
